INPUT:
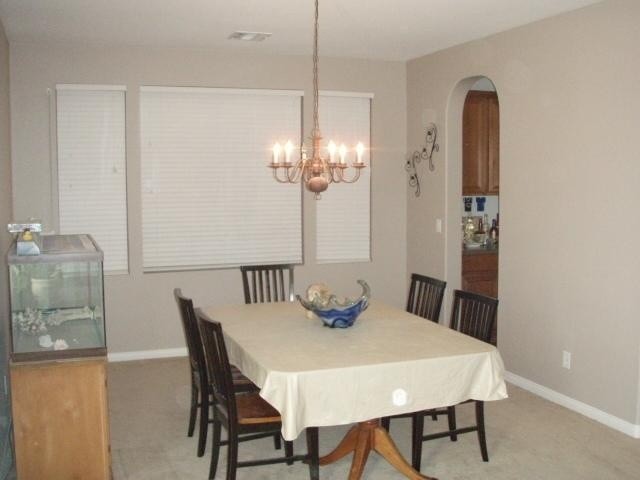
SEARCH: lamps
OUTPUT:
[269,1,368,197]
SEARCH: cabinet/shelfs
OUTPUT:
[464,91,499,195]
[462,245,497,347]
[7,355,114,479]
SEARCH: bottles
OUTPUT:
[463,212,499,248]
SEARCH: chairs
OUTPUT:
[193,306,325,480]
[238,263,296,304]
[381,290,500,471]
[405,271,447,421]
[174,288,263,461]
